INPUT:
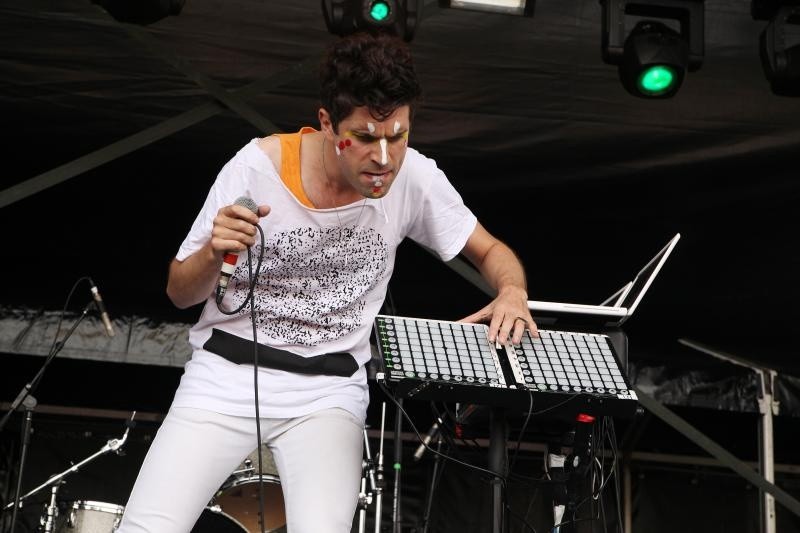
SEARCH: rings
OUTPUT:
[517,317,528,323]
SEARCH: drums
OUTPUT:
[190,506,249,533]
[39,501,127,533]
[208,444,288,533]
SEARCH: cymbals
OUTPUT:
[366,344,384,380]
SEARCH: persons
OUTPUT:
[111,29,540,533]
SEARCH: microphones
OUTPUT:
[90,280,114,337]
[215,196,258,305]
[415,411,449,459]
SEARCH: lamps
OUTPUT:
[599,0,706,99]
[750,0,800,99]
[320,0,423,45]
[437,0,537,19]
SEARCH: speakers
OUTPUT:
[0,403,161,533]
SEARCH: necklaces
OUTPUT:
[321,135,368,266]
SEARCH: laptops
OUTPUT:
[527,232,682,317]
